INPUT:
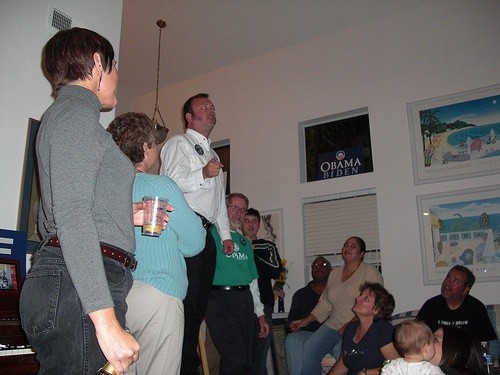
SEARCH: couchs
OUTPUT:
[321,304,500,375]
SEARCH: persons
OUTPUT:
[0,270,9,289]
[105,94,500,375]
[20,27,174,375]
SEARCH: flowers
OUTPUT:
[272,256,294,300]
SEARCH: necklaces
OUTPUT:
[343,264,359,280]
[136,168,144,174]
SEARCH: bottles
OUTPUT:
[478,341,493,375]
[95,363,120,375]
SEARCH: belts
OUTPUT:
[45,236,138,270]
[213,284,250,291]
[195,212,213,231]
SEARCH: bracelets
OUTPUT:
[363,368,367,375]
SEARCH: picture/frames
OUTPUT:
[0,257,23,297]
[416,185,500,286]
[258,207,285,260]
[406,83,500,187]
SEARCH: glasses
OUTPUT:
[312,262,329,267]
[228,205,248,214]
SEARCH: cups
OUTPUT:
[141,195,169,237]
